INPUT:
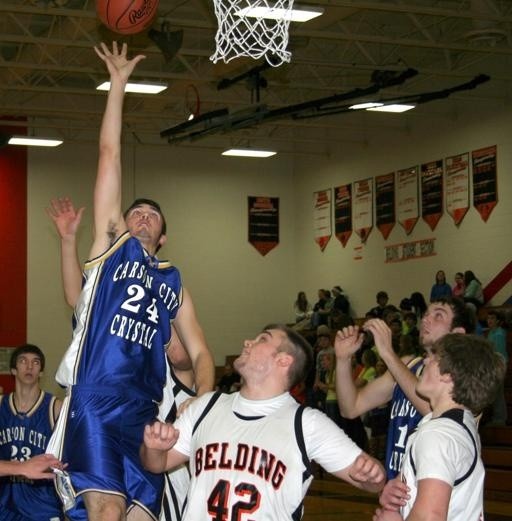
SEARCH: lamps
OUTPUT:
[346,100,417,113]
[231,0,325,23]
[95,79,169,95]
[220,146,279,158]
[7,135,64,148]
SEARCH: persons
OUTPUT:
[44,40,216,521]
[141,323,387,521]
[334,297,476,483]
[217,365,241,394]
[430,270,507,356]
[352,291,428,437]
[0,345,63,520]
[0,453,69,479]
[372,331,507,520]
[48,196,195,520]
[290,285,351,423]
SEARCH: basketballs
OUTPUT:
[96,1,157,34]
